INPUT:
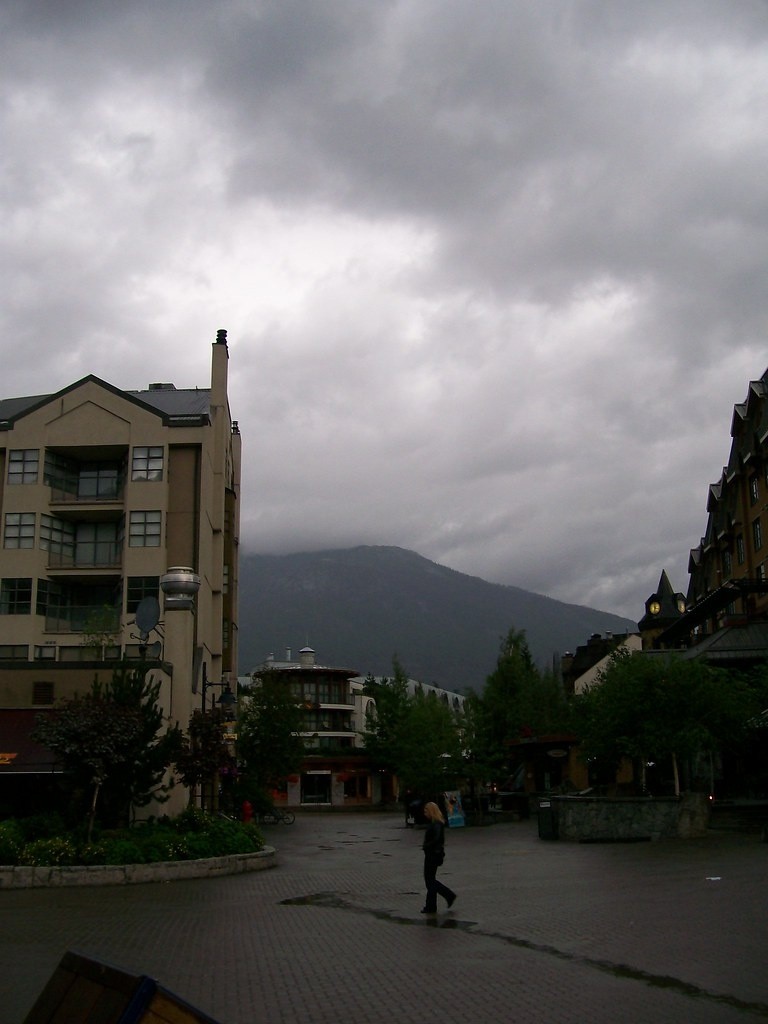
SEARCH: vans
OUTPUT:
[404,766,489,826]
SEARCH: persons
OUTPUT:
[487,790,498,808]
[420,802,457,914]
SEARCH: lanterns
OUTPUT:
[336,772,350,783]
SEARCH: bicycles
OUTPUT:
[263,806,296,825]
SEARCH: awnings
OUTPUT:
[0,708,68,774]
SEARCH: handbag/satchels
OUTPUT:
[407,814,415,824]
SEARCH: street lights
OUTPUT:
[199,662,242,817]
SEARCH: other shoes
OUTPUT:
[421,907,437,913]
[448,895,456,908]
